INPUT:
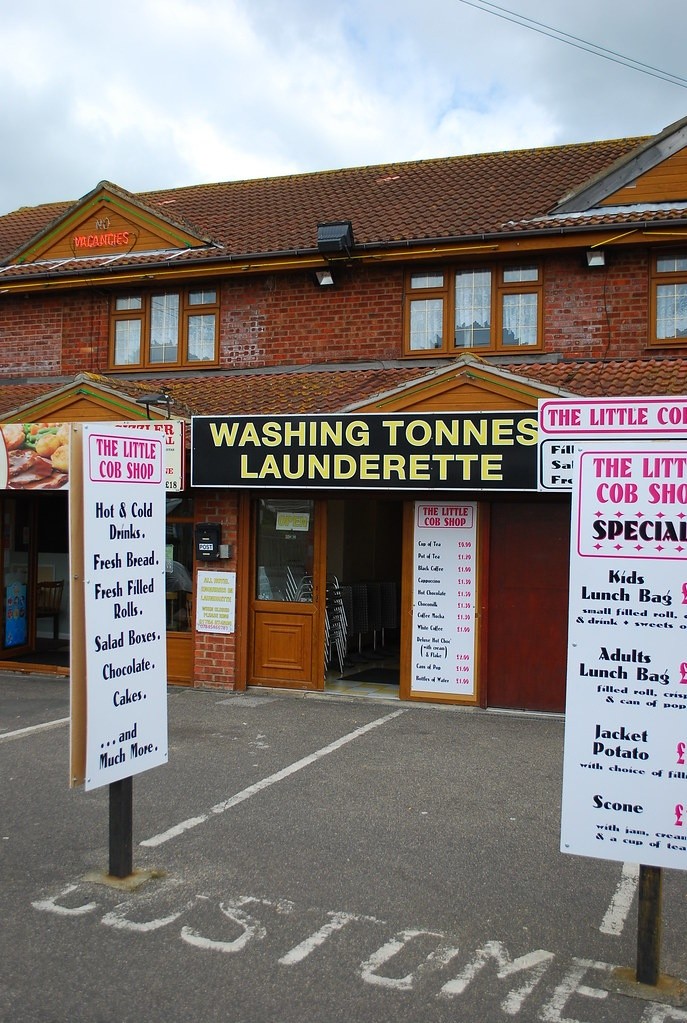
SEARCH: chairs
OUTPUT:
[281,559,349,681]
[166,592,192,632]
[36,579,65,650]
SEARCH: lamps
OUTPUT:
[583,246,610,269]
[316,221,356,259]
[135,386,178,420]
[309,266,339,289]
